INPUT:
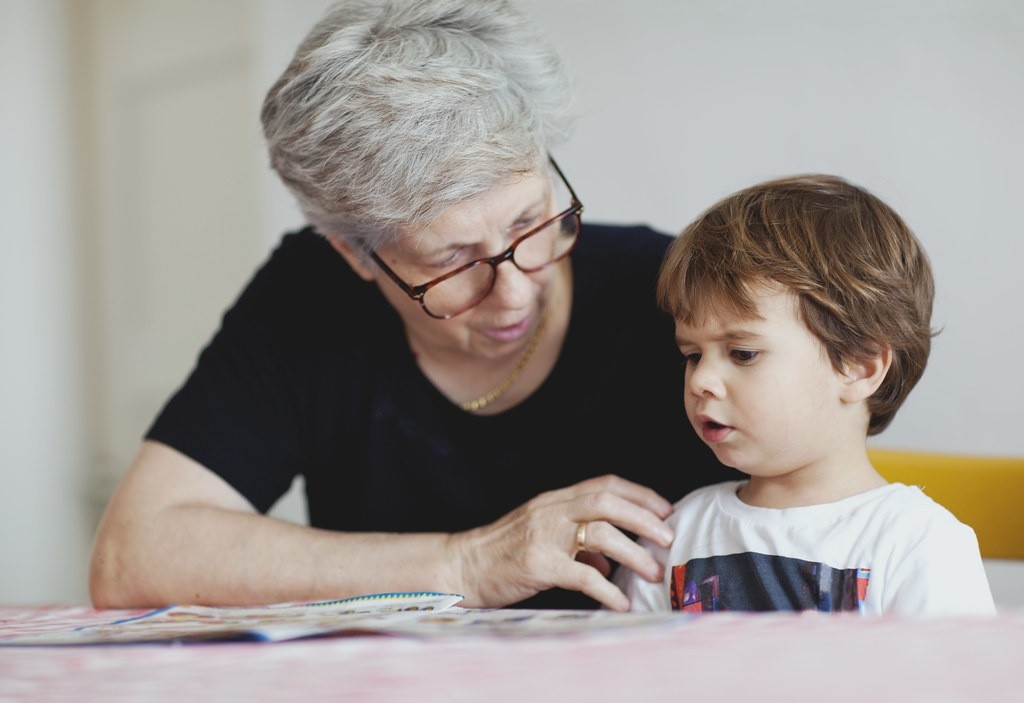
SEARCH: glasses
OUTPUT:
[360,149,585,320]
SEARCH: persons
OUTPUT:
[89,0,751,613]
[603,176,997,616]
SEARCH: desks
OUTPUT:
[0,608,1022,701]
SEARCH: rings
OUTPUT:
[577,521,588,551]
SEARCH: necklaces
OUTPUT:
[459,309,548,410]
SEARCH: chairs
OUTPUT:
[867,447,1024,560]
